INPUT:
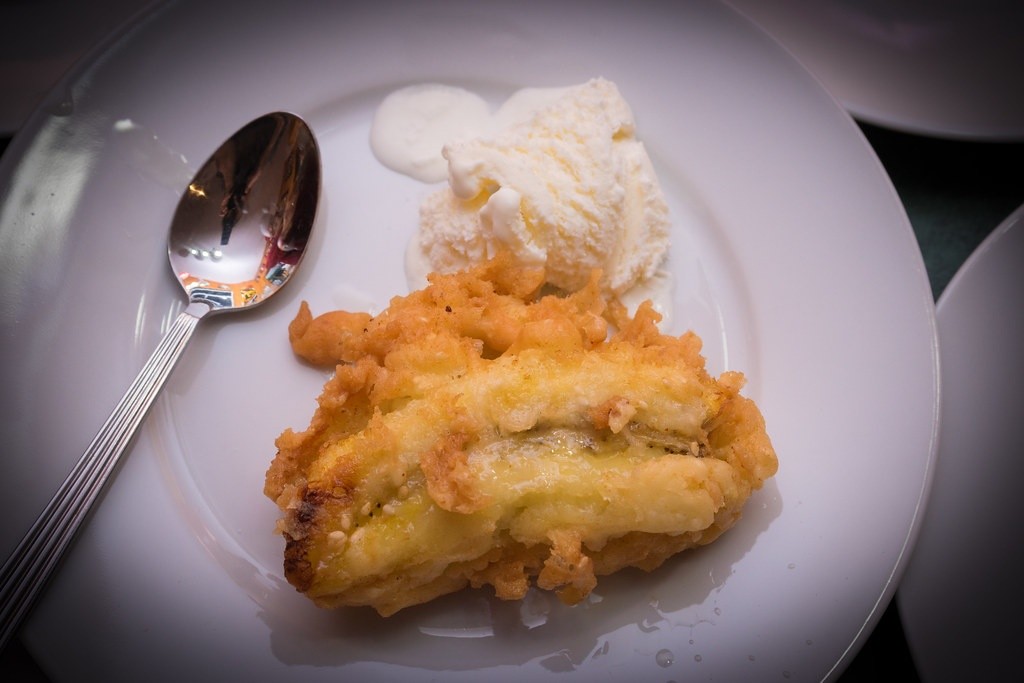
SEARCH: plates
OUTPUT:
[893,202,1024,681]
[730,0,1023,140]
[1,0,941,681]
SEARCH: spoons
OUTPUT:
[3,111,324,648]
